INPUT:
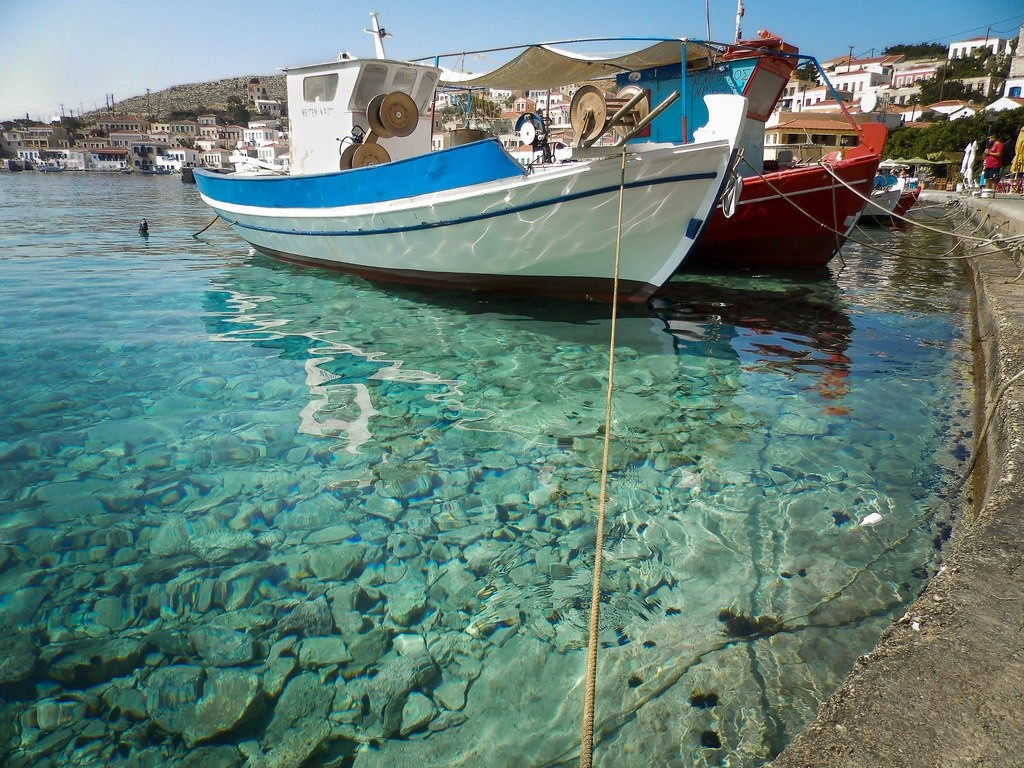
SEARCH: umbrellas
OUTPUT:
[1011,127,1024,180]
[959,140,978,184]
[878,157,959,178]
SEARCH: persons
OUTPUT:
[889,166,899,178]
[899,167,906,178]
[984,133,1004,198]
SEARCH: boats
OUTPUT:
[38,163,65,173]
[139,163,173,175]
[191,0,923,309]
